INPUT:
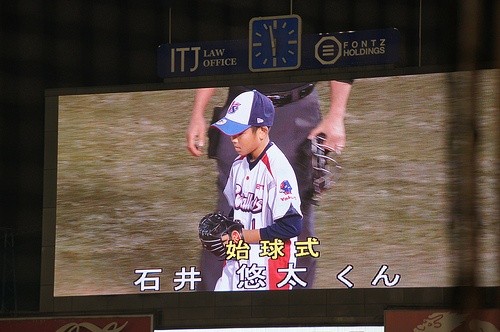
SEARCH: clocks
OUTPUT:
[249,15,302,72]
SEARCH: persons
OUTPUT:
[199,90,303,291]
[186,79,354,291]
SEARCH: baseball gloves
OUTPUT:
[199,213,245,260]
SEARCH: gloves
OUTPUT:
[198,212,244,261]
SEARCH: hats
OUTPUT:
[210,89,275,135]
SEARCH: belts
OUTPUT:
[266,83,315,108]
